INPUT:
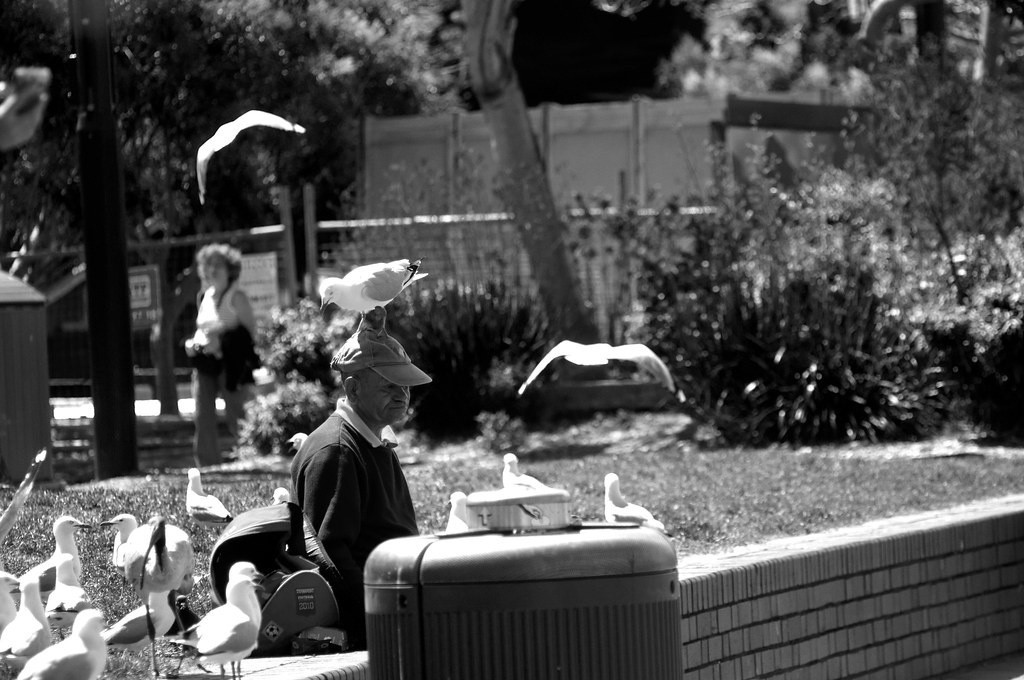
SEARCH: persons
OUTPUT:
[291,328,420,660]
[184,243,264,471]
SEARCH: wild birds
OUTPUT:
[445,491,472,536]
[0,432,344,680]
[318,255,428,333]
[501,453,551,526]
[516,337,676,401]
[603,471,675,540]
[195,109,307,208]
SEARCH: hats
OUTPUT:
[330,328,433,387]
